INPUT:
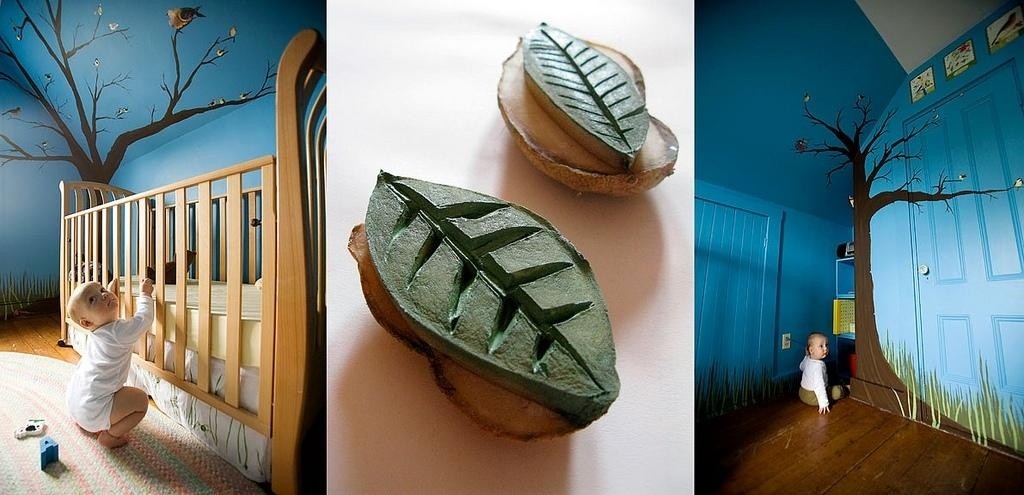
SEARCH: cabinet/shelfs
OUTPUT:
[835,256,857,381]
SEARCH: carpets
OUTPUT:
[0,345,268,495]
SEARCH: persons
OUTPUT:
[796,333,851,416]
[63,276,155,450]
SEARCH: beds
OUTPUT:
[54,26,326,495]
